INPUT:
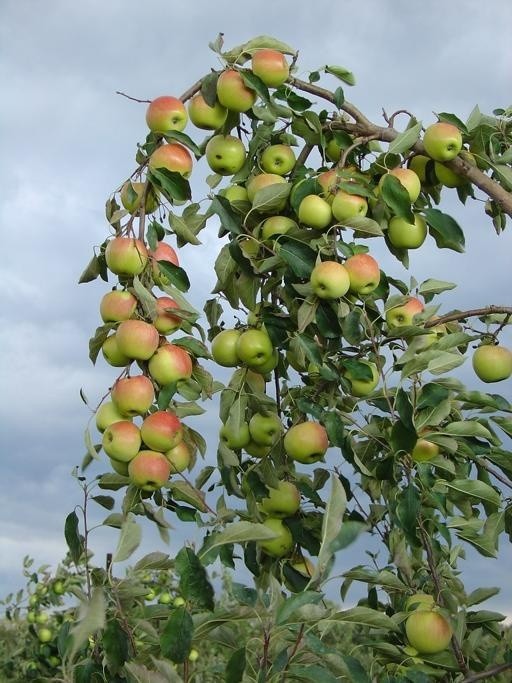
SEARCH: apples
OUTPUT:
[28,50,511,666]
